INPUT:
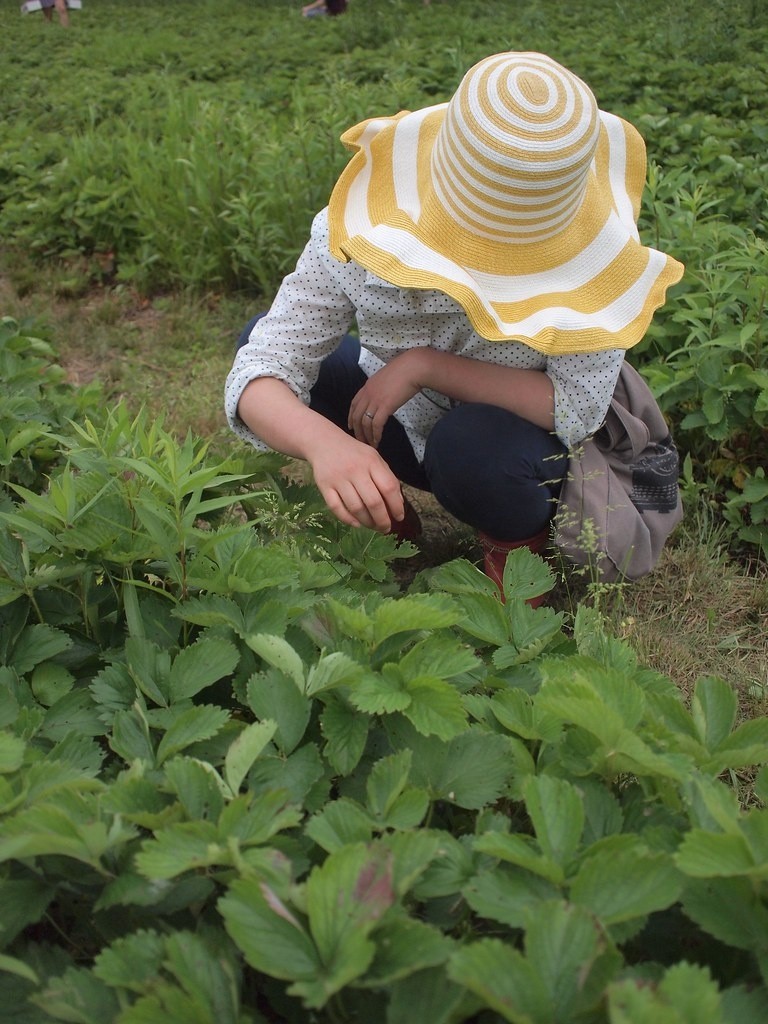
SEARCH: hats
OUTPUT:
[325,46,685,355]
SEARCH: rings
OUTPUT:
[363,410,375,420]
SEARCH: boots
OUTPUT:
[475,525,549,609]
[379,493,422,549]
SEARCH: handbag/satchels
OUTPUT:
[553,362,684,584]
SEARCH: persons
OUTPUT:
[223,56,687,613]
[302,1,348,18]
[39,0,68,27]
